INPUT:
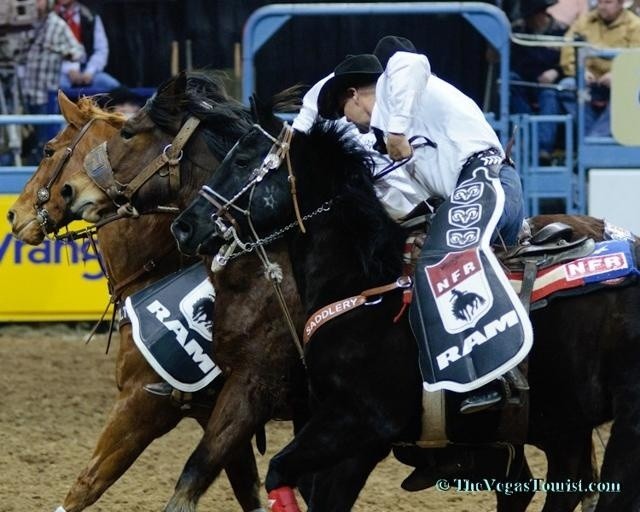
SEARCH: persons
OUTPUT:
[0,1,148,169]
[315,51,535,415]
[293,34,432,228]
[492,1,640,171]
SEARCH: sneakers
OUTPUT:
[539,149,551,166]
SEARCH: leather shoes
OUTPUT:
[401,465,447,491]
[142,382,172,397]
[460,388,503,416]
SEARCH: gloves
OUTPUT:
[387,133,412,161]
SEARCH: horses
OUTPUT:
[7,61,640,512]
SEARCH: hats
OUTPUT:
[317,53,384,120]
[345,34,417,70]
